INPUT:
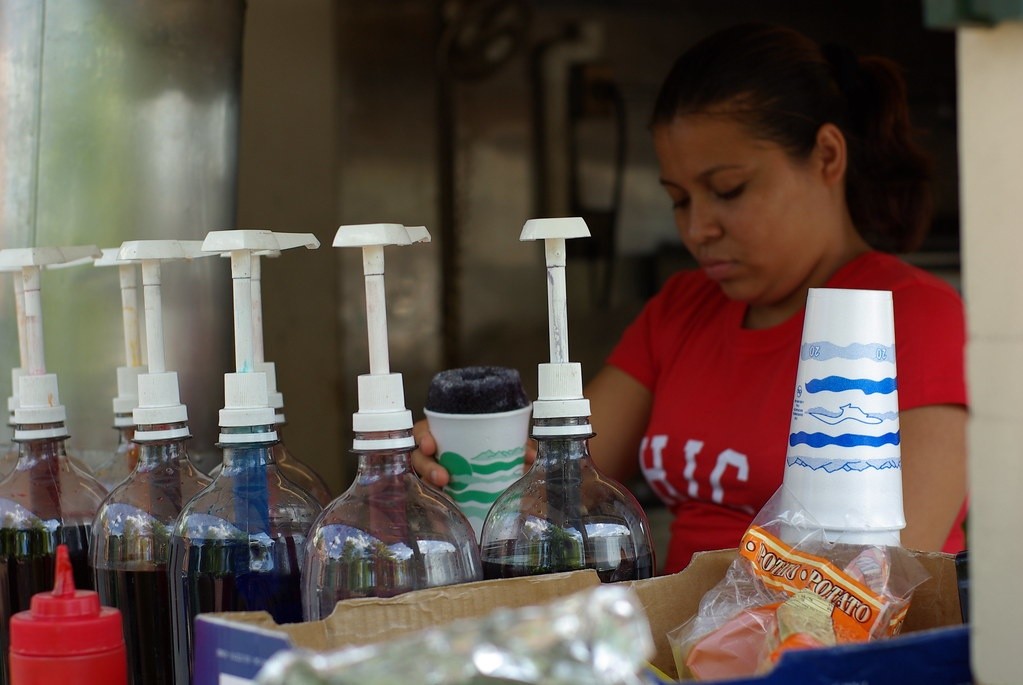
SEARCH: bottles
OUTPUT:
[480,399,654,583]
[206,405,336,507]
[302,414,479,623]
[88,404,235,685]
[0,371,103,483]
[169,394,321,685]
[9,545,127,685]
[0,370,111,685]
[94,397,144,495]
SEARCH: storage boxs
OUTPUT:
[192,542,973,684]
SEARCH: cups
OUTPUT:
[779,287,907,549]
[423,402,533,545]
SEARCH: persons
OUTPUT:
[410,19,966,680]
